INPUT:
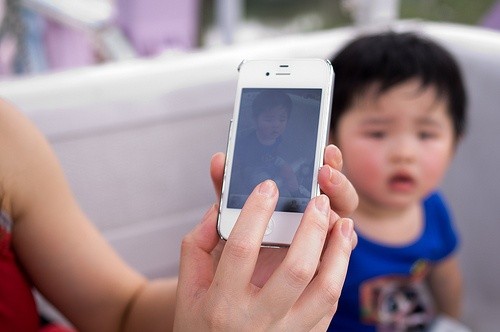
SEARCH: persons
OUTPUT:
[0,91,361,332]
[316,30,474,331]
[229,91,308,199]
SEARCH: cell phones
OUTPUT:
[217,58,335,246]
